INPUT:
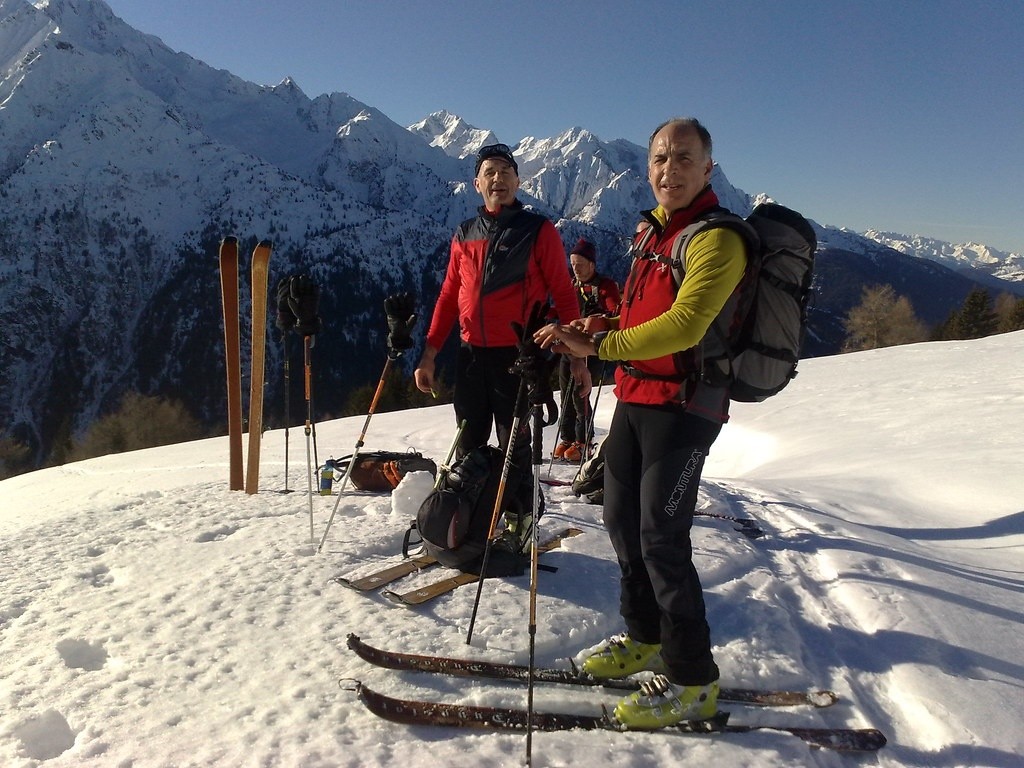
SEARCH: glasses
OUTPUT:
[474,143,514,174]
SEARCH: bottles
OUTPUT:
[320,459,333,496]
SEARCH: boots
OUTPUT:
[492,511,532,556]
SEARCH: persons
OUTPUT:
[534,118,758,731]
[415,143,591,557]
[554,240,623,461]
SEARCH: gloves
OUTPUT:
[508,300,562,383]
[287,275,324,335]
[580,295,600,319]
[383,291,418,350]
[275,275,304,335]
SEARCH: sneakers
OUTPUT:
[615,674,719,730]
[564,440,596,461]
[583,631,663,680]
[553,440,572,458]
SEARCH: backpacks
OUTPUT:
[402,443,546,576]
[630,202,817,403]
[572,434,609,505]
[314,446,437,493]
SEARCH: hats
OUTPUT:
[474,153,518,178]
[569,238,597,265]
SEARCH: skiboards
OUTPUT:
[341,629,888,755]
[536,448,602,467]
[333,501,583,604]
[217,230,271,496]
[535,471,767,544]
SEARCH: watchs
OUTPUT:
[593,330,608,353]
